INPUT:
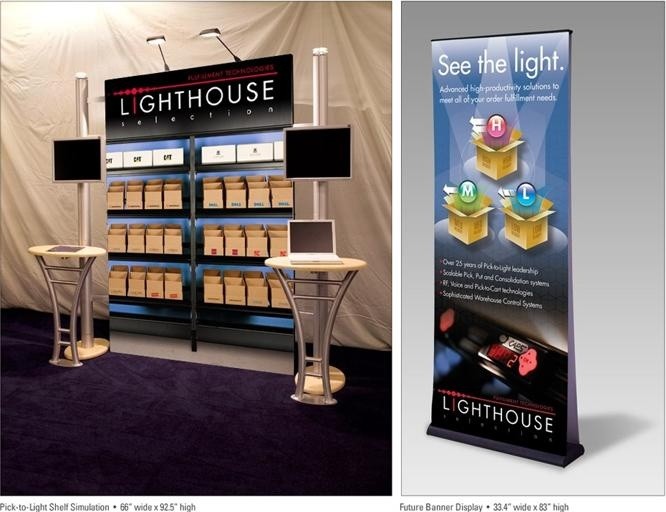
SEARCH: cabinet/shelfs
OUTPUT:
[105,126,295,352]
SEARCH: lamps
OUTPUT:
[146,35,169,72]
[199,28,241,61]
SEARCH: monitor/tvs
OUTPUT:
[283,123,353,181]
[52,136,105,183]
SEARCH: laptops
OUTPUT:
[287,219,344,265]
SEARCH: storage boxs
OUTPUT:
[470,128,526,181]
[442,192,495,245]
[106,141,295,309]
[498,194,557,251]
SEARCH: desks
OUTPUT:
[264,255,367,406]
[29,244,107,368]
